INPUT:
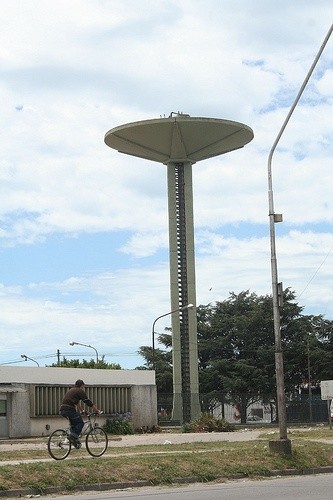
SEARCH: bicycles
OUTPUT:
[47,410,108,460]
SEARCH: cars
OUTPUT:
[235,415,265,424]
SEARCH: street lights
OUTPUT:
[69,341,99,364]
[152,303,195,368]
[20,353,39,366]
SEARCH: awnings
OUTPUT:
[0,385,27,393]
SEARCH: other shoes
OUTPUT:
[71,440,79,449]
[70,435,81,444]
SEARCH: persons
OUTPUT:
[60,379,101,449]
